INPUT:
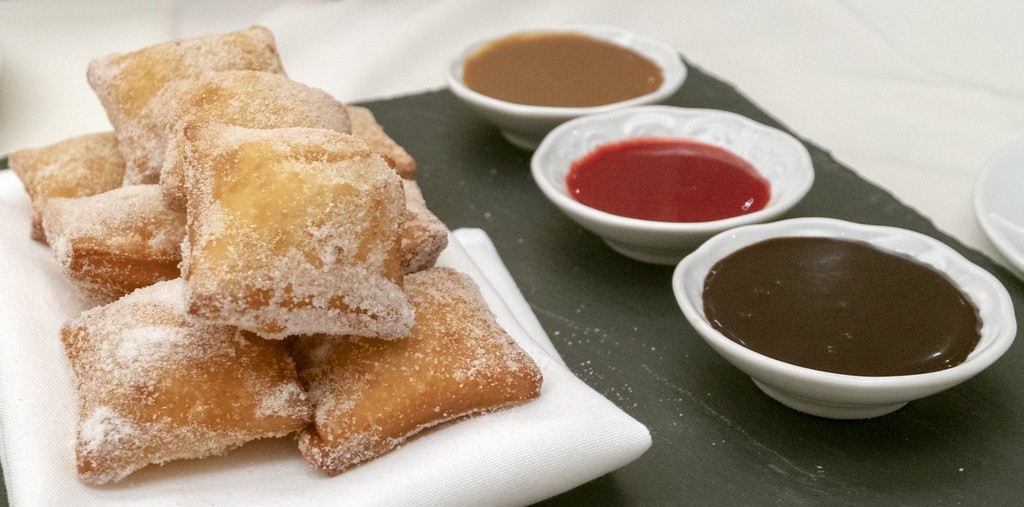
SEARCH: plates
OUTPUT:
[974,135,1023,270]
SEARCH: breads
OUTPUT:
[9,24,543,472]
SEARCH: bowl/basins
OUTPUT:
[672,217,1017,418]
[441,23,688,153]
[529,105,815,265]
